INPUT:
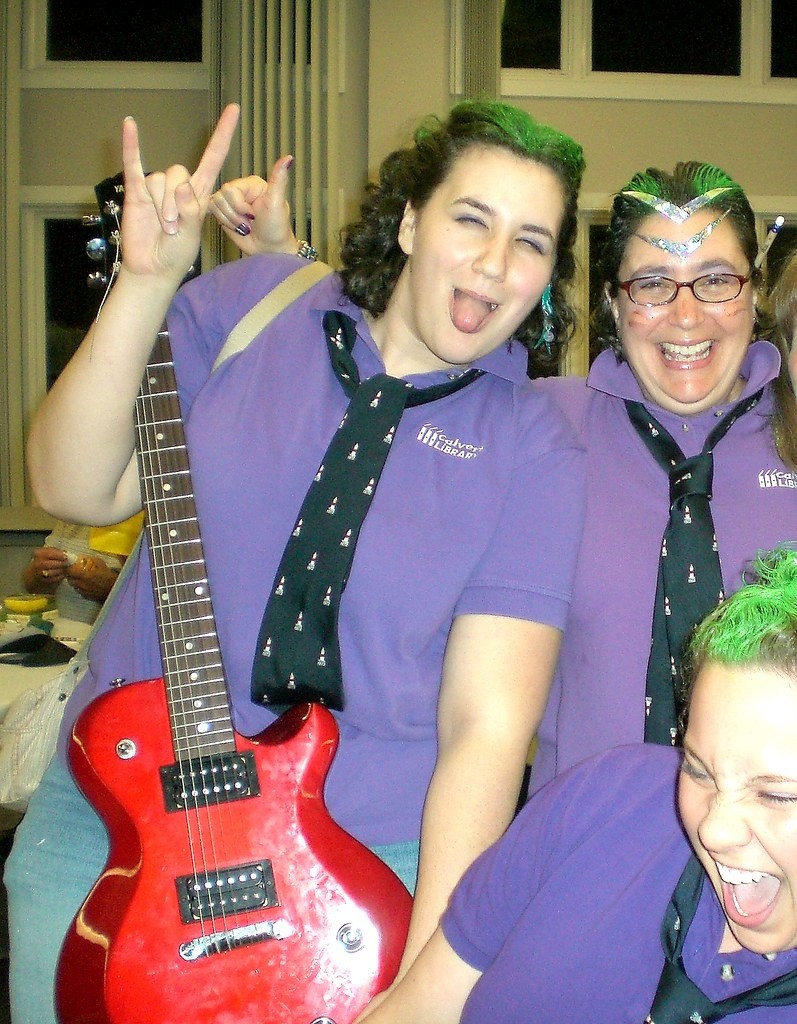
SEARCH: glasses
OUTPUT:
[614,269,754,307]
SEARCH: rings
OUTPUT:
[42,570,49,577]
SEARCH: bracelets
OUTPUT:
[298,239,319,262]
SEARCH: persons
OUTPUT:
[2,102,586,1024]
[760,250,797,474]
[0,509,146,830]
[206,154,797,1024]
[360,552,797,1024]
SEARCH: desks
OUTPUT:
[0,620,93,833]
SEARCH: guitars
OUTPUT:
[51,167,414,1024]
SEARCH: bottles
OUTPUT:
[68,552,97,572]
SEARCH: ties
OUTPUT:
[624,388,764,751]
[251,309,489,717]
[644,853,797,1024]
